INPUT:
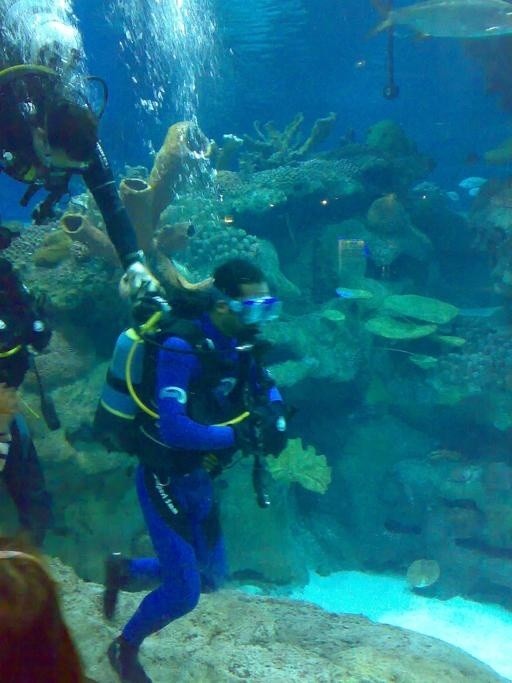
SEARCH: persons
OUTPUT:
[0,11,168,299]
[0,539,99,683]
[101,255,291,682]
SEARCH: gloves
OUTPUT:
[118,259,166,308]
[231,400,292,459]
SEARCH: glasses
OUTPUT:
[42,161,91,176]
[219,294,288,330]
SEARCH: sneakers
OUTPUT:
[105,632,153,682]
[100,549,129,624]
